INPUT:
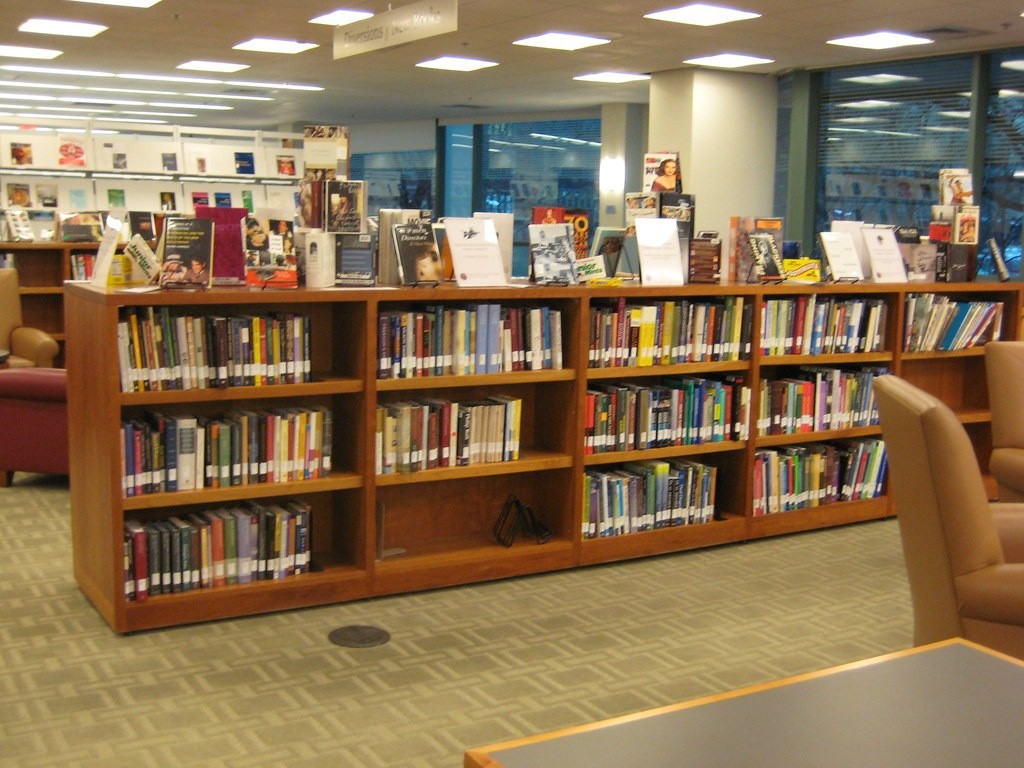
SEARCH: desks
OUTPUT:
[464,638,1024,768]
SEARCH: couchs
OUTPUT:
[872,374,1024,658]
[0,268,60,368]
[983,341,1024,502]
[0,367,70,488]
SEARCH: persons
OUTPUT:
[181,255,210,285]
[651,158,676,194]
[271,222,294,239]
[333,190,350,227]
[162,255,186,283]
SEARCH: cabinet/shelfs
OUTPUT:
[0,124,1024,636]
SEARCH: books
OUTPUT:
[760,364,894,436]
[0,126,981,289]
[117,305,311,392]
[584,374,750,454]
[754,440,888,517]
[373,390,522,474]
[377,302,563,379]
[903,291,1005,352]
[119,402,331,497]
[583,458,718,541]
[761,293,890,357]
[590,297,753,368]
[123,500,313,603]
